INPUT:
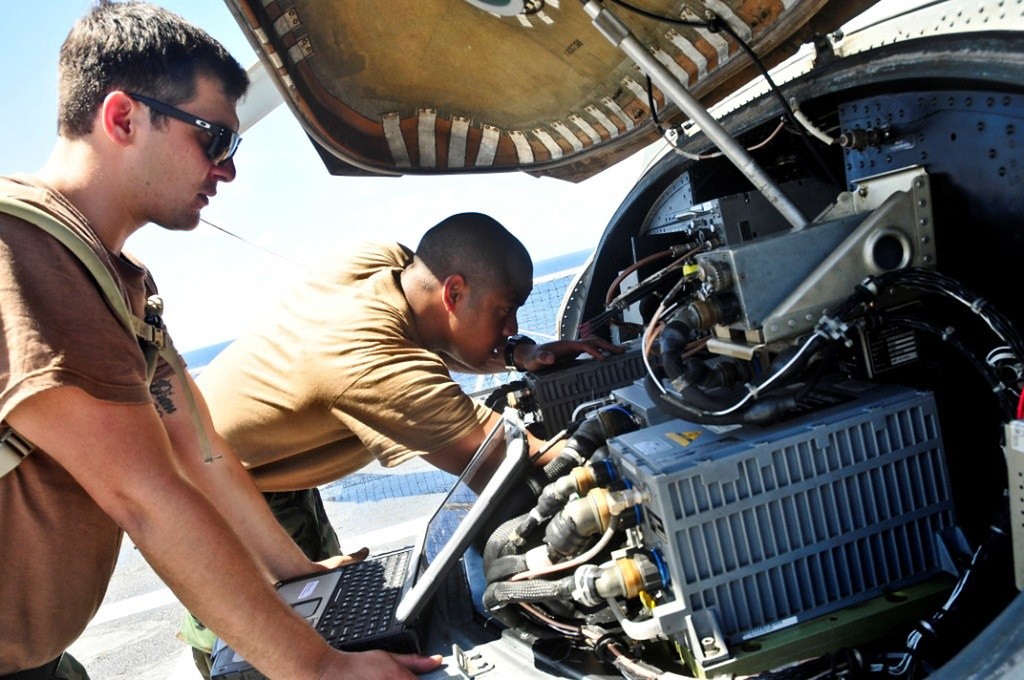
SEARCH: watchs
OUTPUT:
[505,334,537,372]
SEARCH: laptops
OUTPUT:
[210,409,527,680]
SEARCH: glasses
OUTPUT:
[98,91,242,167]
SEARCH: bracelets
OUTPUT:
[177,212,629,680]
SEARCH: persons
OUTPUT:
[0,1,445,678]
